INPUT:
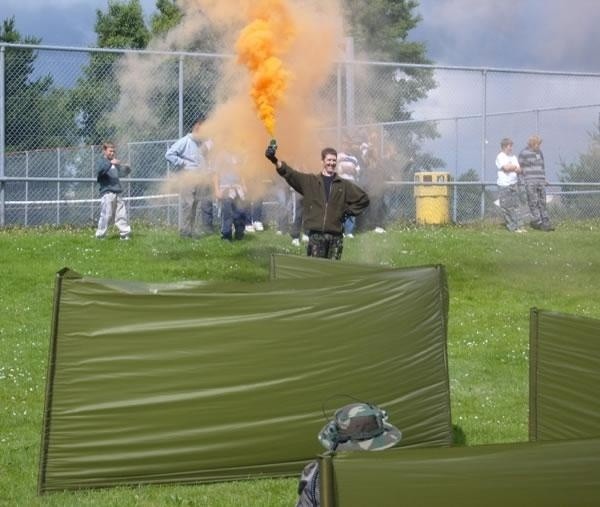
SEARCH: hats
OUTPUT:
[317,403,401,453]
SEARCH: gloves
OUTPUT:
[266,148,278,162]
[341,210,352,222]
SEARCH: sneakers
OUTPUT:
[342,232,353,239]
[291,234,309,246]
[369,227,384,233]
[513,227,527,233]
[538,223,555,231]
[245,221,263,234]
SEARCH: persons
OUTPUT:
[296,402,402,504]
[164,117,217,240]
[202,128,396,246]
[92,140,135,241]
[263,140,372,262]
[496,136,527,233]
[516,134,557,232]
